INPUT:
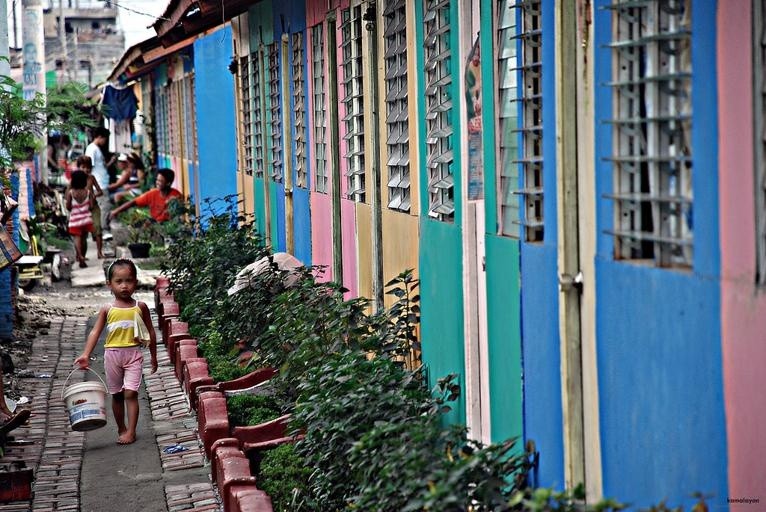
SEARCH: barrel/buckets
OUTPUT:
[60,366,110,433]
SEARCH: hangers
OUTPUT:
[108,78,129,91]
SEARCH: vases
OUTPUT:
[126,242,152,260]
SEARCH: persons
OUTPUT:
[46,127,186,267]
[72,258,160,445]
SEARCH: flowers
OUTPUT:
[116,209,156,244]
